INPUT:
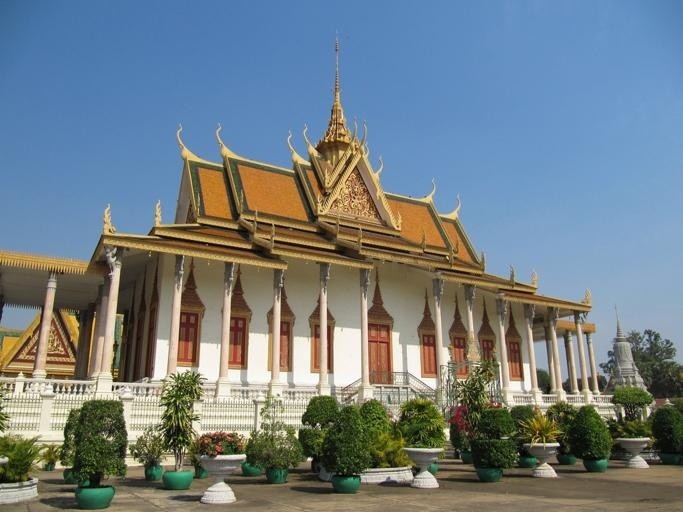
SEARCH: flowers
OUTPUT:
[192,429,245,457]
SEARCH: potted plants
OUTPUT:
[394,397,446,488]
[60,409,82,484]
[299,395,445,482]
[73,400,128,511]
[129,424,168,481]
[241,430,265,476]
[322,421,369,494]
[451,360,683,469]
[259,423,300,484]
[37,443,60,471]
[189,441,208,479]
[157,370,208,490]
[570,406,611,473]
[474,440,516,481]
[519,413,561,477]
